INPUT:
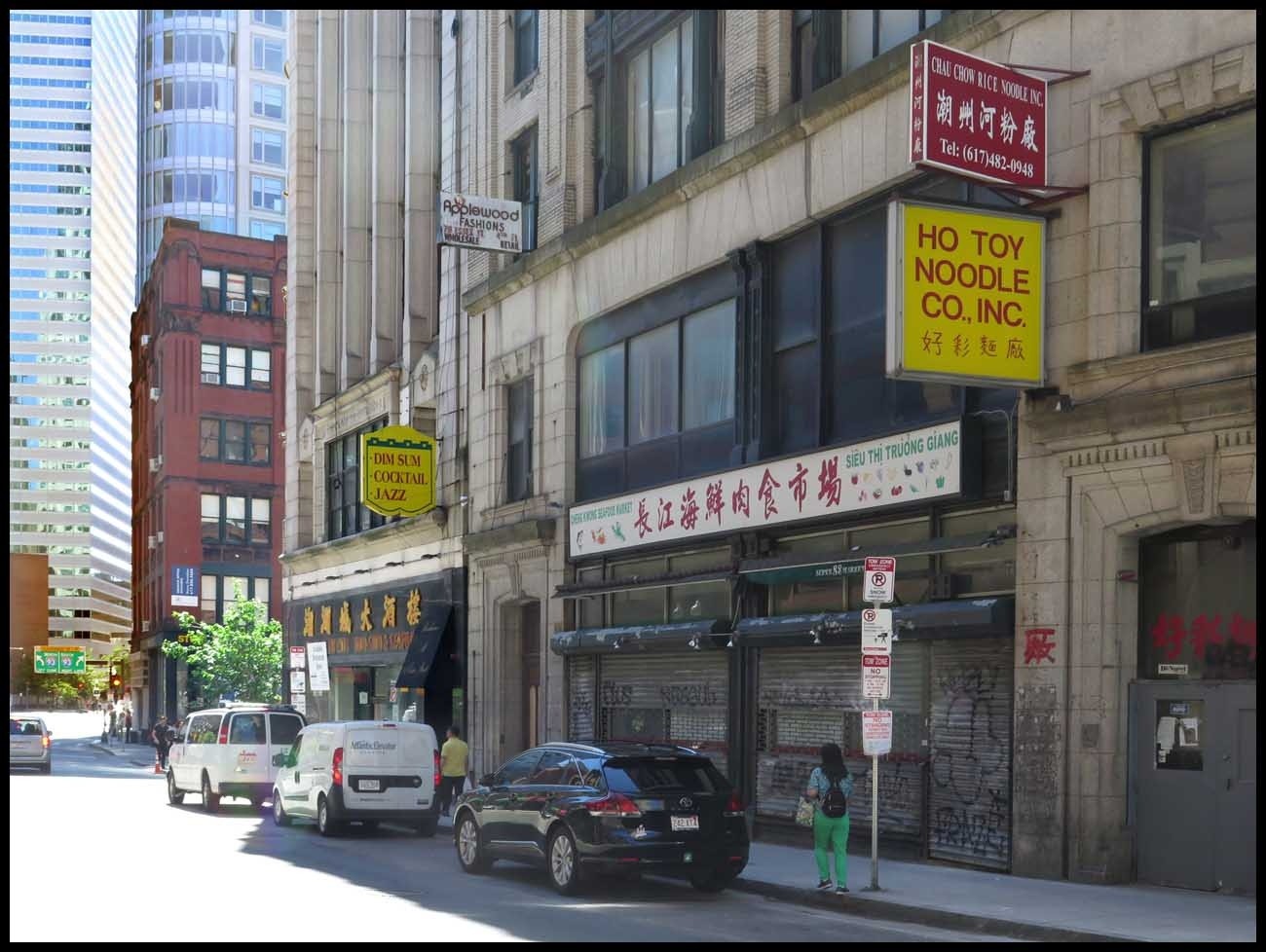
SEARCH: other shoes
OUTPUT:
[835,886,850,895]
[441,810,450,817]
[818,879,832,889]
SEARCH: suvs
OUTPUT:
[452,740,750,898]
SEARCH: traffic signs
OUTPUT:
[34,645,59,674]
[58,646,86,675]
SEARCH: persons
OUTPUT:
[807,743,851,895]
[440,726,469,818]
[84,696,185,772]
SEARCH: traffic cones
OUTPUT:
[155,749,164,773]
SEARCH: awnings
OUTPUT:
[394,600,455,693]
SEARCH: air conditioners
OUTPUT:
[150,388,160,399]
[141,335,151,346]
[205,374,219,383]
[140,621,150,632]
[148,531,163,549]
[228,299,247,312]
[149,453,163,473]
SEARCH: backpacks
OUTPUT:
[820,775,847,818]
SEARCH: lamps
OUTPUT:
[614,636,624,648]
[811,620,827,644]
[688,632,702,651]
[892,623,903,642]
[727,631,740,648]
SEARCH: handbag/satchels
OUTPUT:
[794,795,815,826]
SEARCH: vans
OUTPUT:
[9,713,53,773]
[271,719,442,836]
[167,702,308,814]
